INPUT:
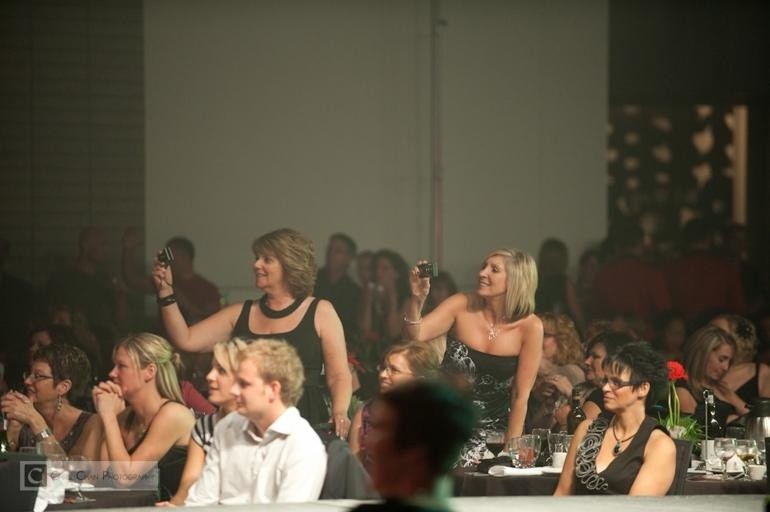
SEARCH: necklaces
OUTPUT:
[488,322,500,342]
[612,417,637,455]
[258,290,309,319]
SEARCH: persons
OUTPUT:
[91,332,195,501]
[723,224,766,314]
[650,308,686,361]
[357,248,411,363]
[151,227,352,449]
[41,226,126,329]
[426,270,456,307]
[570,249,601,310]
[347,338,438,475]
[526,313,585,434]
[709,313,770,409]
[670,326,751,432]
[553,342,677,496]
[169,337,254,505]
[121,227,219,324]
[154,338,328,508]
[313,231,360,345]
[535,239,581,322]
[11,325,64,393]
[1,342,102,478]
[590,222,671,323]
[548,331,633,435]
[404,246,543,468]
[344,376,476,512]
[665,219,745,324]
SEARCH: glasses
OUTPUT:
[600,376,634,391]
[376,362,416,376]
[22,371,56,384]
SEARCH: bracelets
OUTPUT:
[153,292,178,307]
[32,426,52,442]
[403,313,423,325]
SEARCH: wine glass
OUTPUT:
[68,455,97,504]
[714,438,738,480]
[734,439,757,482]
[46,453,67,503]
[486,430,506,469]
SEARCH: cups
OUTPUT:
[748,464,766,480]
[509,427,573,468]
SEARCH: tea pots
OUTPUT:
[750,415,770,478]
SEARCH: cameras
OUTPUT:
[417,261,440,280]
[158,246,176,271]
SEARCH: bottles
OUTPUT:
[567,387,586,434]
